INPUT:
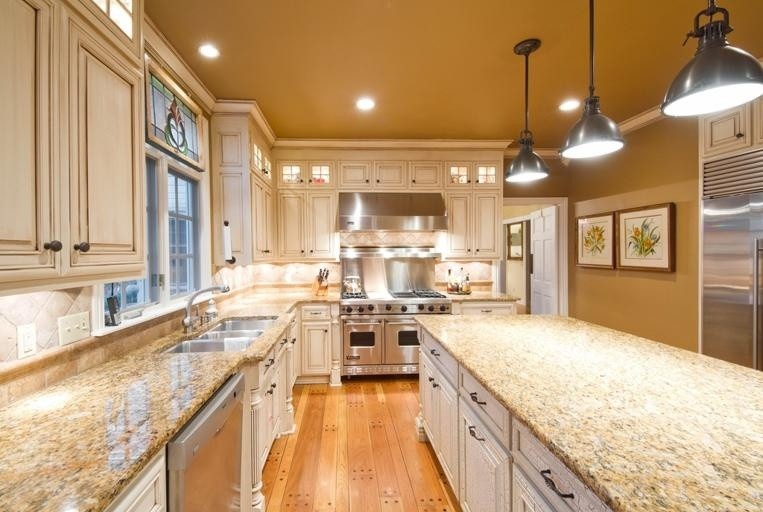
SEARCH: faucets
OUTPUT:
[183,284,230,336]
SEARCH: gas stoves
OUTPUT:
[337,284,453,314]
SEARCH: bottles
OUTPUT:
[446,264,472,292]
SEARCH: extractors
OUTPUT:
[336,189,448,234]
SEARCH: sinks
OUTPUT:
[195,332,261,338]
[162,339,255,353]
[211,319,279,330]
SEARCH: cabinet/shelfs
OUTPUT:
[209,113,272,187]
[460,364,512,512]
[275,159,337,189]
[461,301,516,315]
[213,173,275,262]
[250,299,340,474]
[408,161,443,191]
[437,192,503,262]
[699,94,763,159]
[275,190,340,263]
[417,325,459,505]
[0,0,149,294]
[338,161,407,192]
[511,414,611,512]
[443,160,500,189]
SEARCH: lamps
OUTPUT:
[658,1,763,117]
[504,37,549,185]
[560,0,626,159]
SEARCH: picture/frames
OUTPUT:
[507,222,523,261]
[573,210,614,270]
[614,201,676,273]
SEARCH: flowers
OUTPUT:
[584,224,605,256]
[626,218,661,258]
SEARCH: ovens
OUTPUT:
[339,311,421,377]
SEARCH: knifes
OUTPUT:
[318,268,330,282]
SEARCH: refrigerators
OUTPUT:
[700,146,763,371]
